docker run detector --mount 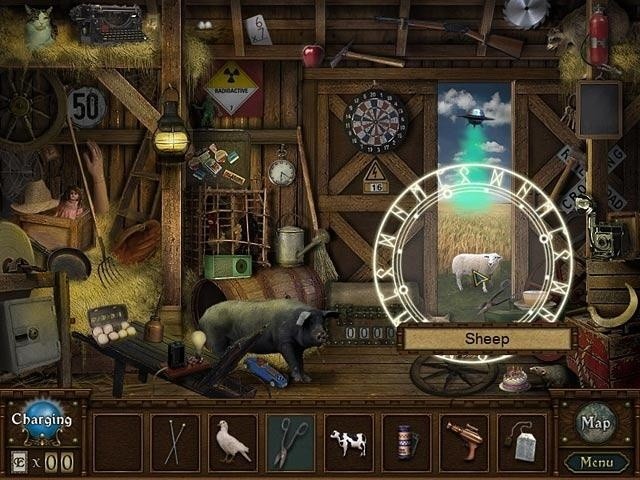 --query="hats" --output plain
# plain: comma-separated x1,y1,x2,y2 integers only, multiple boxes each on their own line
9,179,60,215
